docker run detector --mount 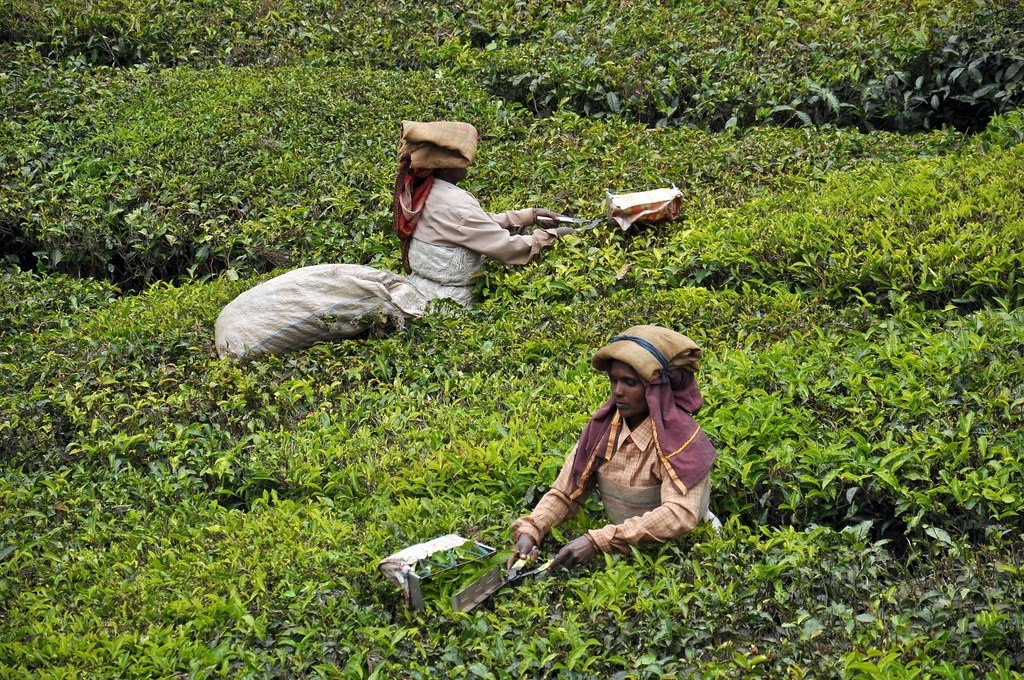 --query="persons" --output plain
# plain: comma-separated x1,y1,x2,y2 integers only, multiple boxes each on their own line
393,120,577,312
506,325,725,581
